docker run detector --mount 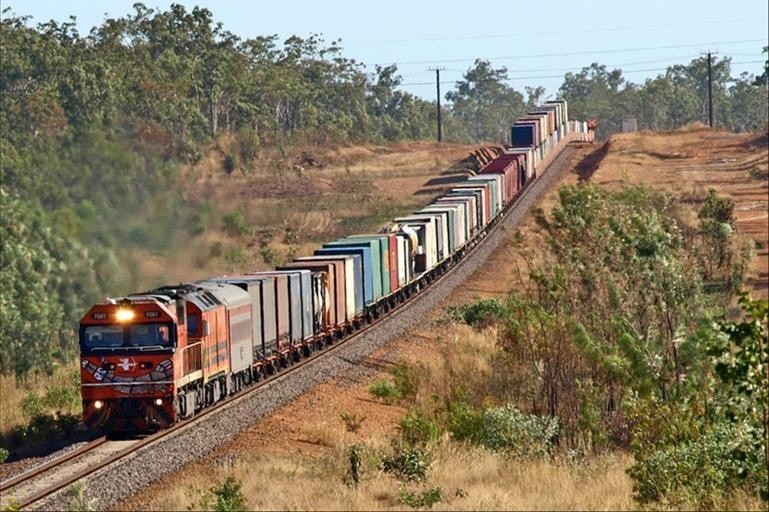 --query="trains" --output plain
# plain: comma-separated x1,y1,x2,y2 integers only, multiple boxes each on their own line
77,98,598,438
